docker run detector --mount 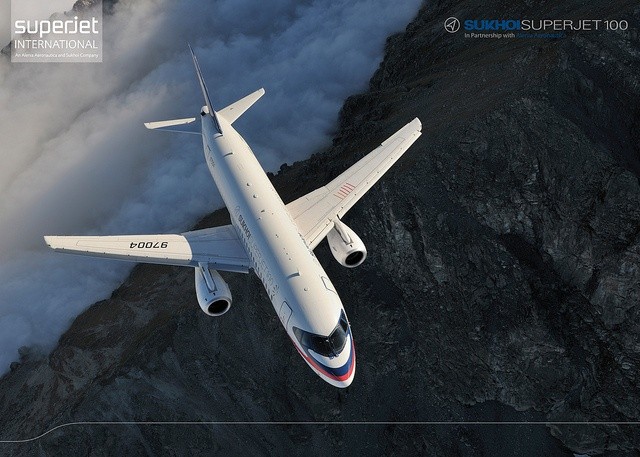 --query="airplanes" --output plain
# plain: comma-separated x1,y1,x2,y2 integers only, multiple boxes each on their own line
42,43,423,390
